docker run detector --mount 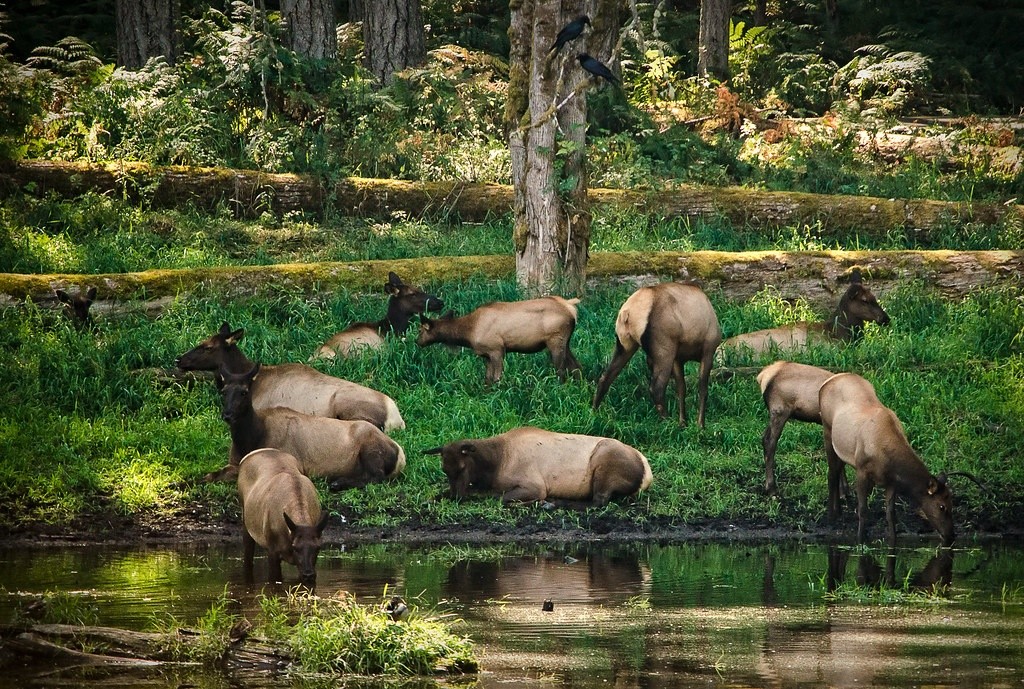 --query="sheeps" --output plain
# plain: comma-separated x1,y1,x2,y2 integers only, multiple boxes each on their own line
757,361,956,547
309,271,446,366
592,282,722,427
176,322,407,583
712,269,892,381
415,295,583,385
421,427,654,511
